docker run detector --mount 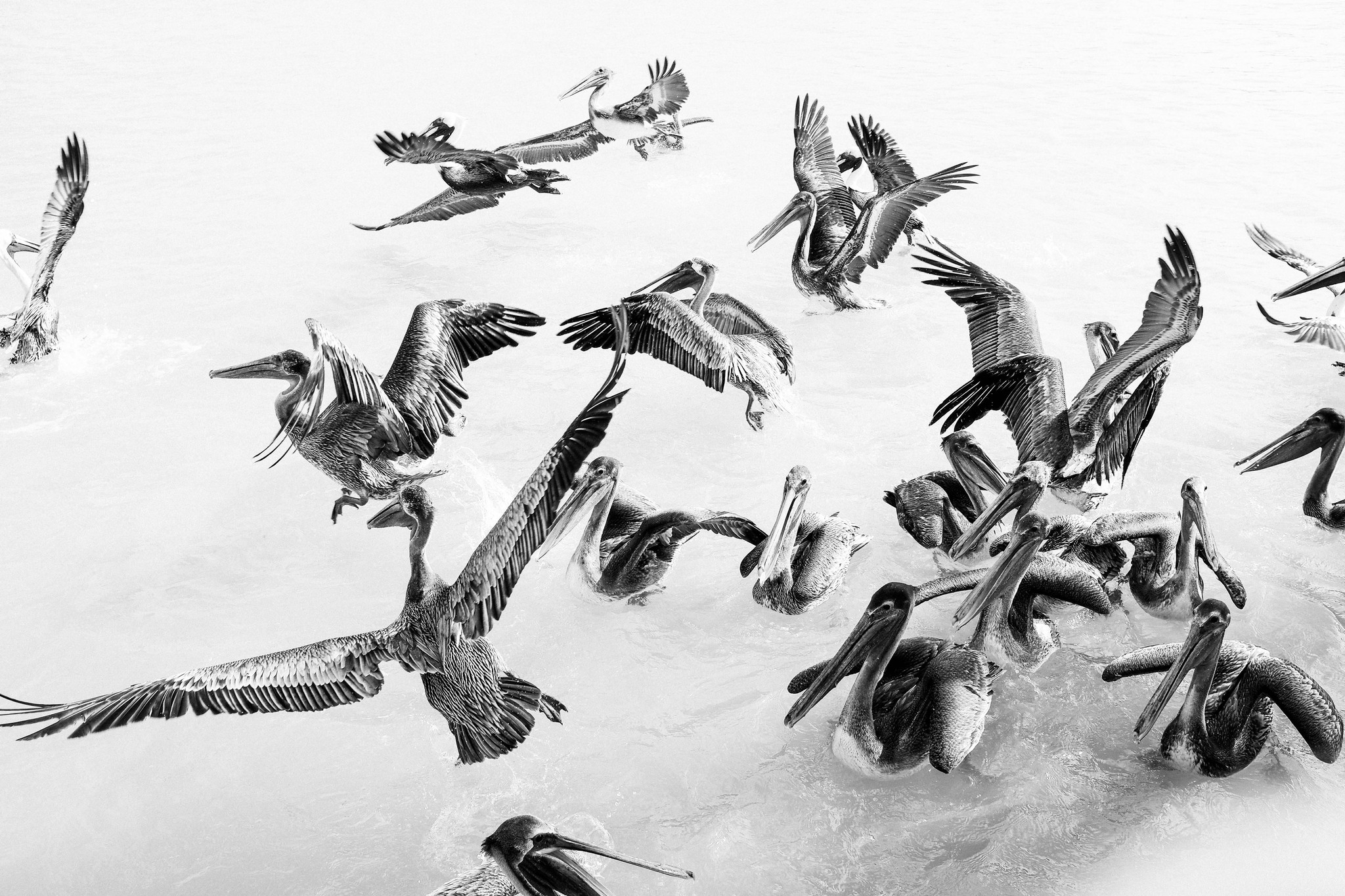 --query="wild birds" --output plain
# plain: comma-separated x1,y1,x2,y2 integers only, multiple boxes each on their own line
738,464,876,615
0,130,91,369
741,91,981,316
1100,599,1344,779
535,456,768,606
881,221,1344,676
846,111,935,247
782,581,1005,777
2,299,632,769
349,115,572,231
209,297,546,526
490,55,713,164
420,814,695,896
555,259,796,431
